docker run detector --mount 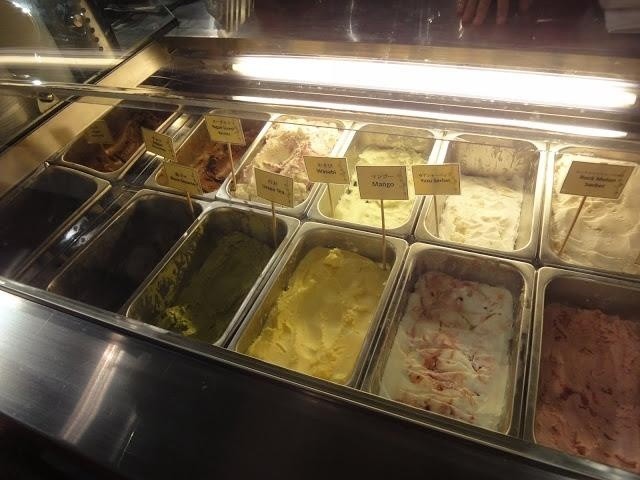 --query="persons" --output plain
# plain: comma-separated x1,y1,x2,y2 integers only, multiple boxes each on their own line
455,1,533,29
